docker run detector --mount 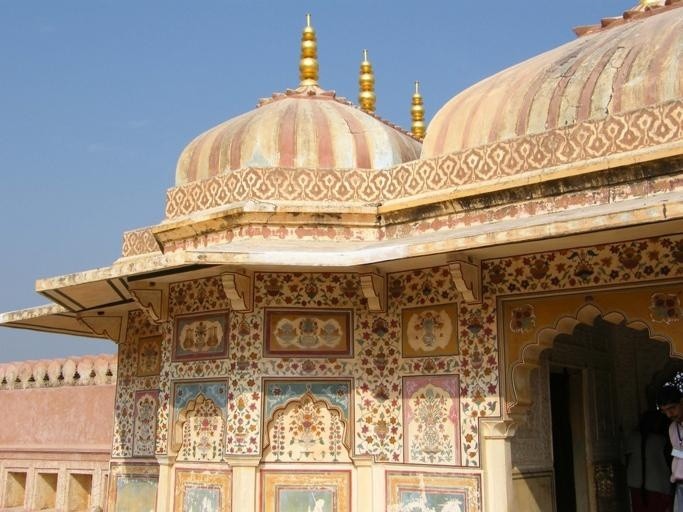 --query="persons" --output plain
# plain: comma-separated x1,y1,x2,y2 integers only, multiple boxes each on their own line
622,410,674,511
656,383,683,512
